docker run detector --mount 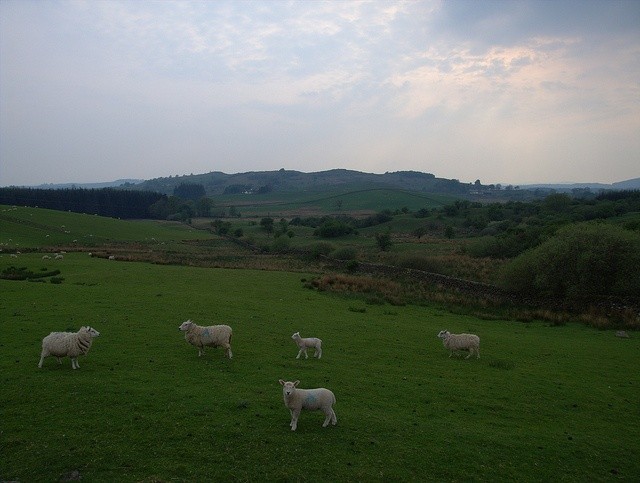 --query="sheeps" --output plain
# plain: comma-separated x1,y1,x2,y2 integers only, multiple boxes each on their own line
37,326,100,370
109,255,115,261
437,329,481,360
178,319,233,360
291,332,322,359
54,255,64,262
42,255,48,261
278,379,337,431
